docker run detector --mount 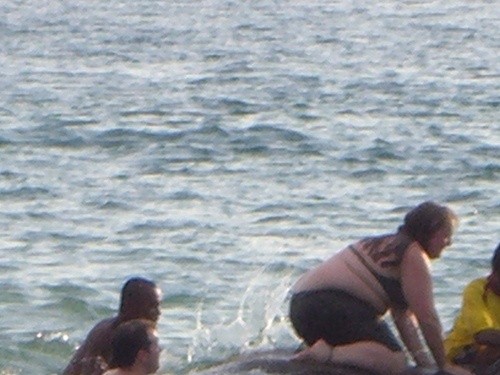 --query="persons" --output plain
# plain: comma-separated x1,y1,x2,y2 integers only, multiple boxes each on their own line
100,319,161,375
445,243,500,375
63,278,162,375
290,201,478,375
